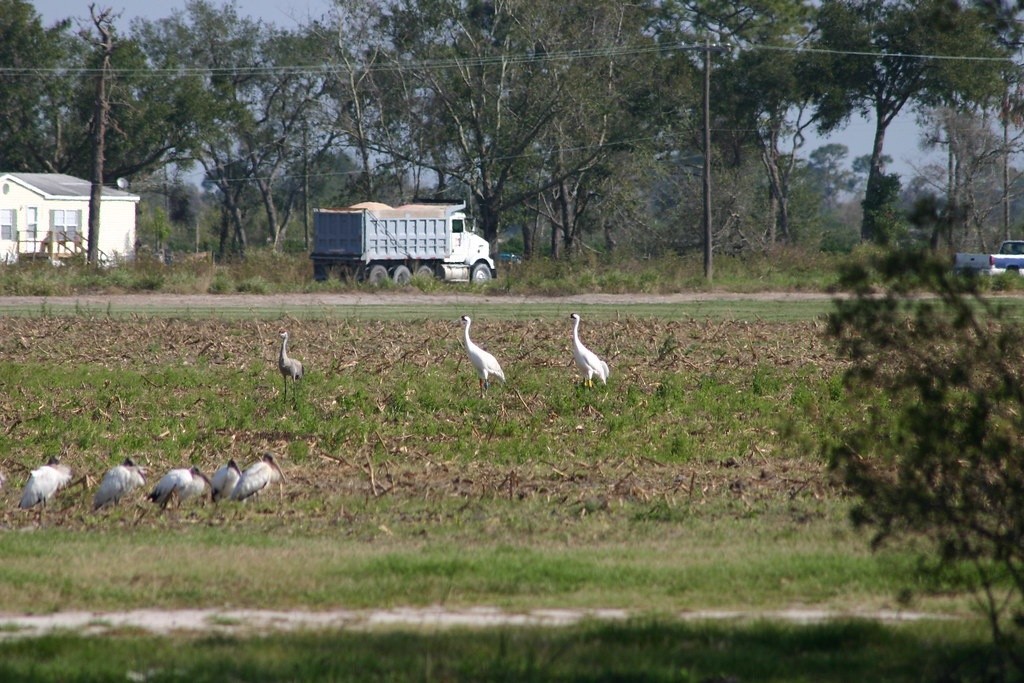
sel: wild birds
[90,452,288,517]
[570,313,610,391]
[452,315,506,398]
[17,460,72,523]
[278,328,304,403]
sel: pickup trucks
[951,238,1024,292]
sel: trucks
[308,196,498,293]
